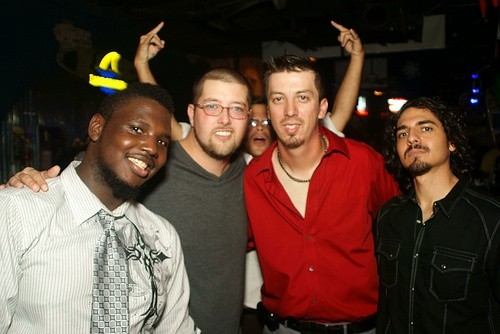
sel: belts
[277,317,375,334]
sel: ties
[90,209,129,334]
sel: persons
[372,98,500,334]
[243,54,402,334]
[0,80,201,334]
[4,66,256,334]
[133,20,365,334]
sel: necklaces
[277,132,327,183]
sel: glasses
[248,118,270,127]
[195,102,252,120]
